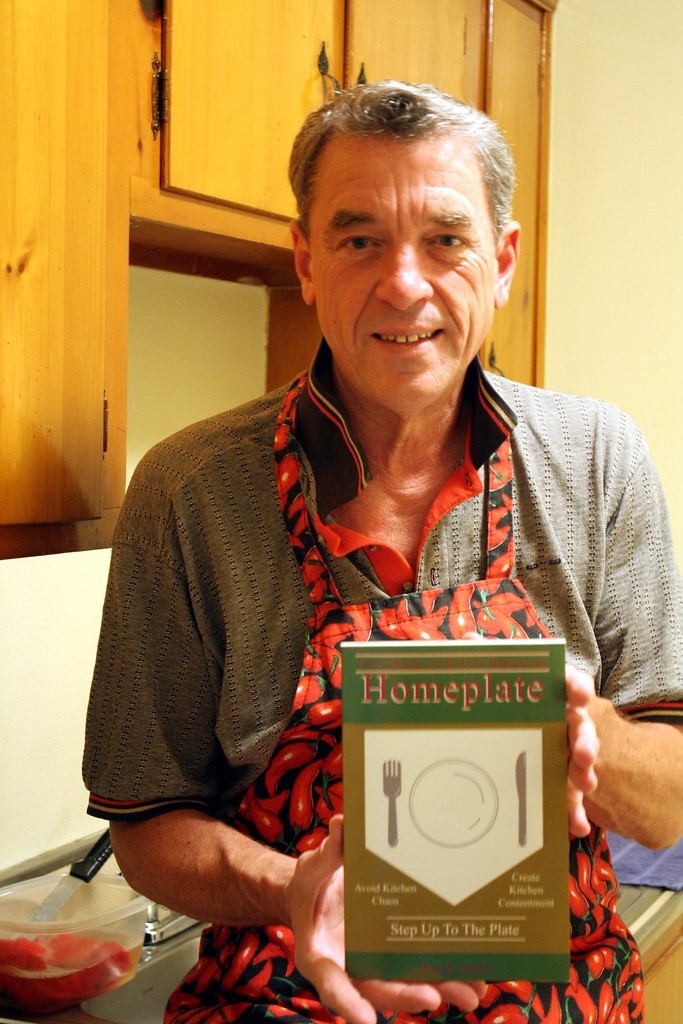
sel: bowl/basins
[0,873,152,1014]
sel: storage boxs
[0,869,154,1011]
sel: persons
[82,83,683,1024]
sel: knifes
[12,828,112,942]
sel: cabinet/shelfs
[630,910,683,1024]
[0,0,557,559]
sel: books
[340,638,571,983]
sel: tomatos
[0,932,133,1008]
